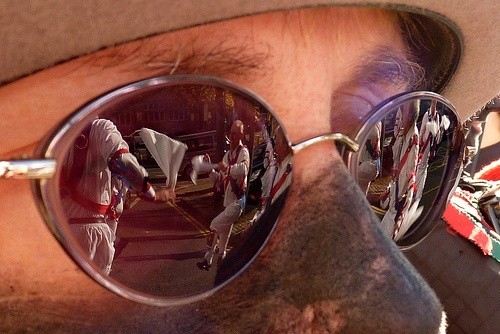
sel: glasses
[1,73,468,308]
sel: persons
[0,0,500,334]
[379,99,420,243]
[414,99,444,201]
[59,114,177,276]
[197,120,250,273]
[268,125,293,206]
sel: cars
[172,130,223,176]
[123,130,163,162]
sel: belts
[68,217,105,224]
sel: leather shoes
[197,260,211,272]
